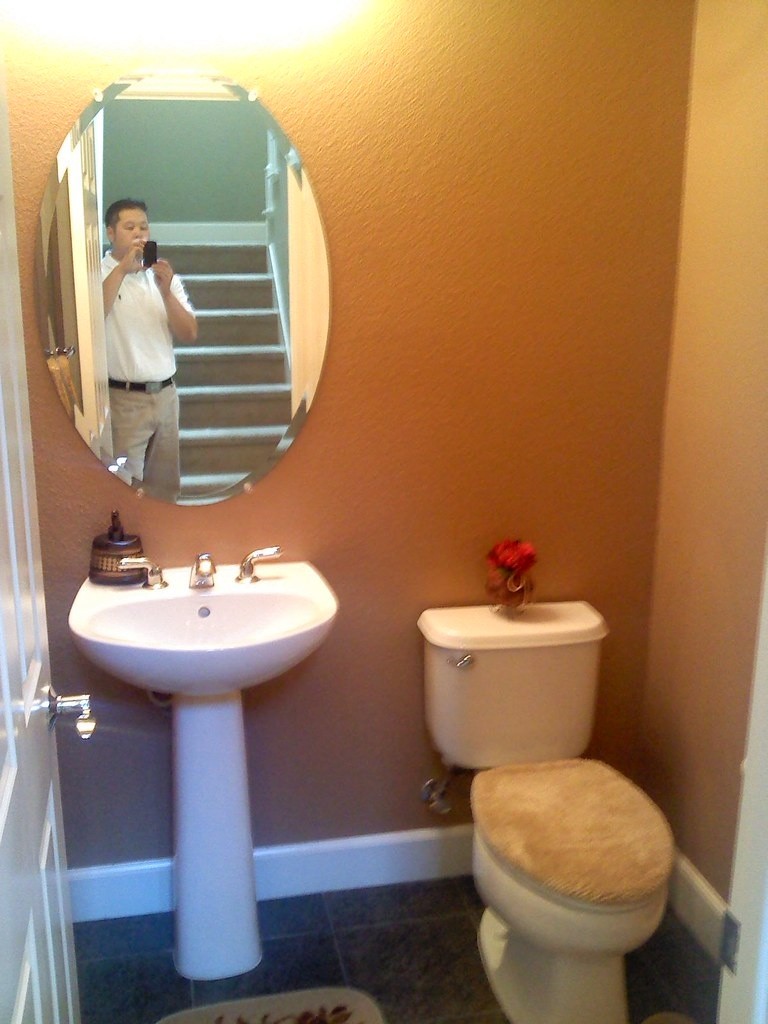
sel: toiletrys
[88,508,148,586]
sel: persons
[102,199,198,487]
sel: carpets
[153,985,387,1023]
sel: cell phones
[143,241,158,268]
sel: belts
[108,378,171,393]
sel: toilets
[414,598,678,1023]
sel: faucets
[189,552,217,589]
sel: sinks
[67,560,339,698]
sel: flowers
[487,540,535,606]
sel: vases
[485,576,535,620]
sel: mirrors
[33,72,335,506]
